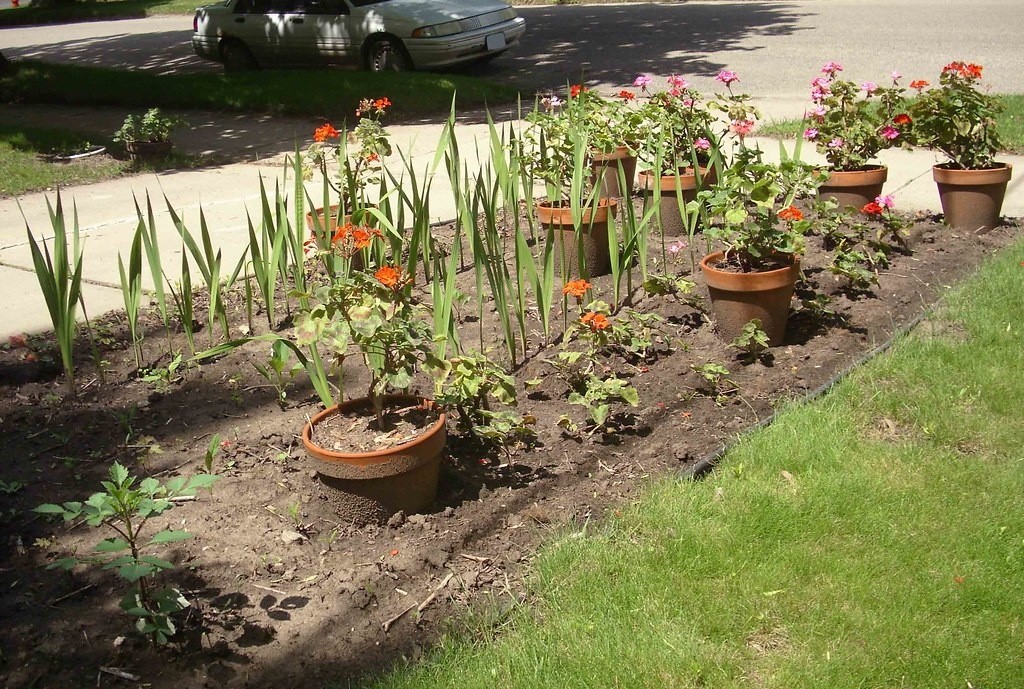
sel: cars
[191,0,528,73]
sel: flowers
[894,60,1008,167]
[801,61,903,167]
[502,66,755,196]
[304,223,450,424]
[306,97,392,199]
[685,165,813,265]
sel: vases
[306,203,378,272]
[302,395,448,514]
[932,161,1013,235]
[636,166,711,237]
[536,200,621,278]
[700,249,800,352]
[690,159,724,191]
[812,165,889,216]
[581,142,639,196]
